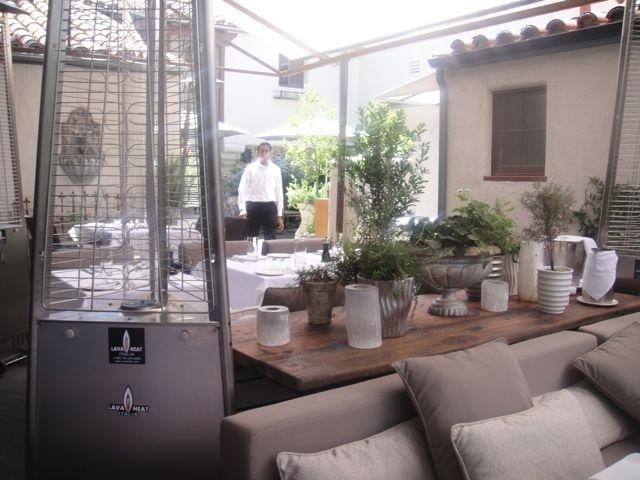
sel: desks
[69,217,202,265]
[218,293,639,391]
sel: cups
[100,246,113,273]
[246,237,258,257]
[293,237,308,267]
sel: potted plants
[286,174,317,238]
[402,187,520,316]
[536,177,575,315]
[300,266,340,326]
[160,153,184,225]
[349,158,429,338]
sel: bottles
[321,241,330,263]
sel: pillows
[448,388,607,480]
[275,415,435,479]
[391,337,534,480]
[572,321,639,426]
[525,375,635,451]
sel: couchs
[218,309,640,480]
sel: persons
[237,142,286,240]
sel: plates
[236,254,267,262]
[268,253,290,258]
[257,268,283,277]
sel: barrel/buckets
[543,239,589,287]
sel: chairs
[179,238,347,307]
[197,214,250,241]
